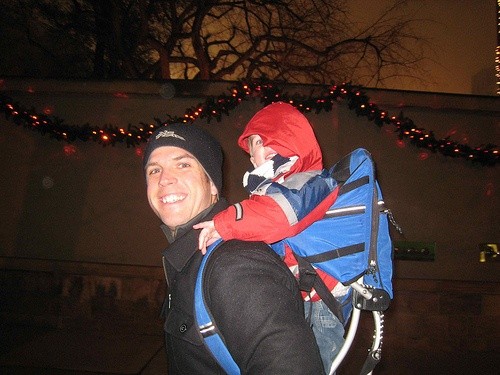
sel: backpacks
[286,148,394,312]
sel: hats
[143,123,224,200]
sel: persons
[142,122,326,375]
[192,101,353,375]
